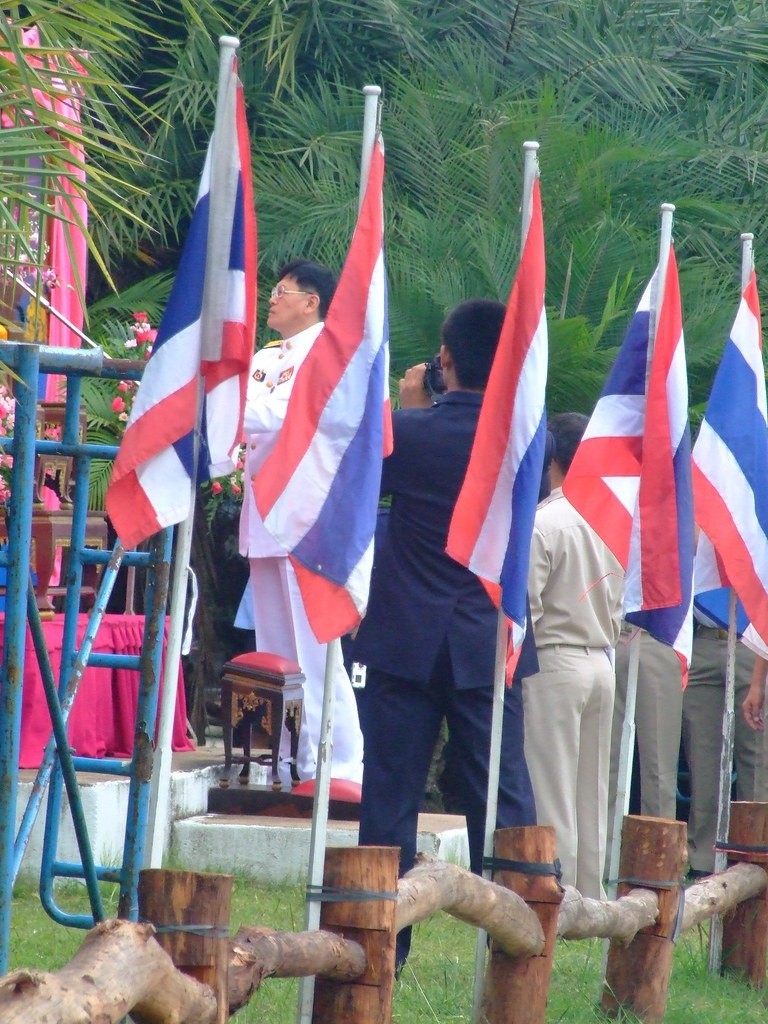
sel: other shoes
[686,866,711,880]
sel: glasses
[271,286,313,299]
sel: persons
[354,301,540,977]
[236,261,365,788]
[613,620,768,877]
[521,412,626,901]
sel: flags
[106,59,257,550]
[251,134,394,643]
[693,271,768,656]
[563,245,693,690]
[444,175,549,688]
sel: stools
[220,652,307,792]
[33,401,88,511]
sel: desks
[0,612,198,771]
[0,511,108,622]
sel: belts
[697,626,728,641]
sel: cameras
[423,354,446,395]
